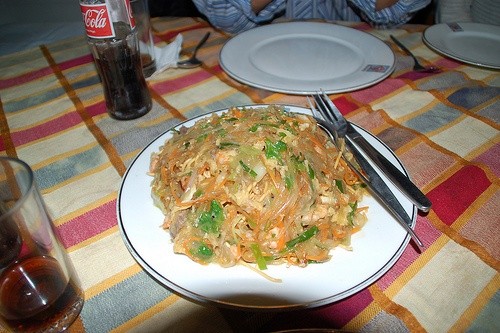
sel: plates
[218,21,397,97]
[423,21,500,70]
[116,102,418,313]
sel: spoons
[176,31,210,69]
[312,116,425,248]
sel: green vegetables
[171,111,367,270]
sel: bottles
[80,0,153,120]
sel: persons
[192,0,431,36]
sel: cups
[128,0,157,79]
[0,155,84,333]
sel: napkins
[138,33,183,81]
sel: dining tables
[0,15,500,333]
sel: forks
[389,34,441,73]
[306,87,432,213]
